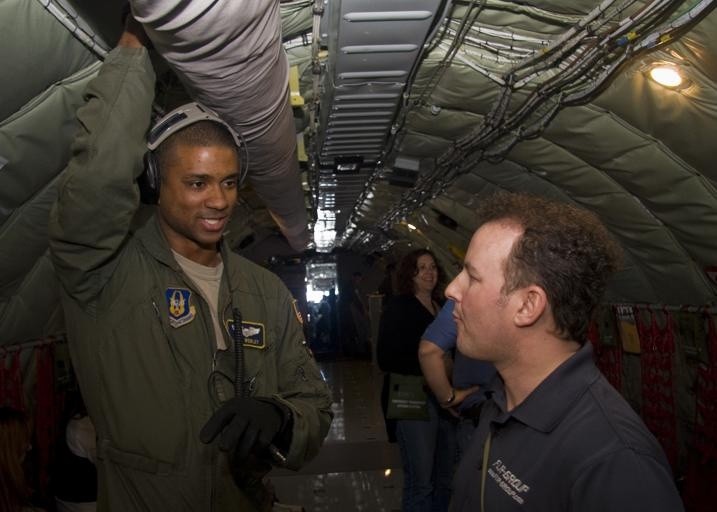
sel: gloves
[198,395,281,471]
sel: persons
[52,384,99,511]
[340,270,364,356]
[417,296,500,455]
[445,186,683,509]
[374,248,461,511]
[304,280,337,340]
[45,14,334,510]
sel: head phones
[138,101,250,206]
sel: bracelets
[439,388,456,405]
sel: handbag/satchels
[385,371,432,421]
